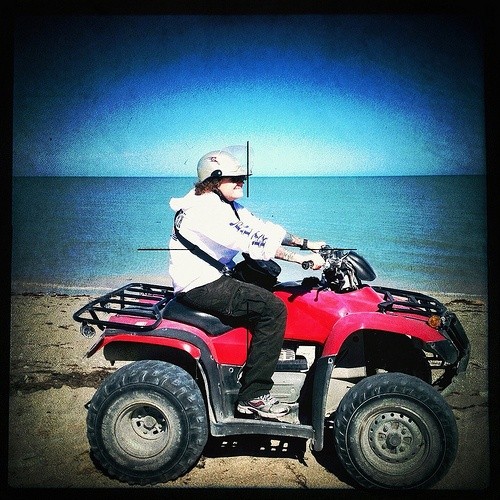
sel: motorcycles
[73,242,472,491]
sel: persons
[168,150,328,419]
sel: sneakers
[237,393,291,419]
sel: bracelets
[302,237,308,249]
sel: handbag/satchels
[174,201,282,289]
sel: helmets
[197,150,252,184]
